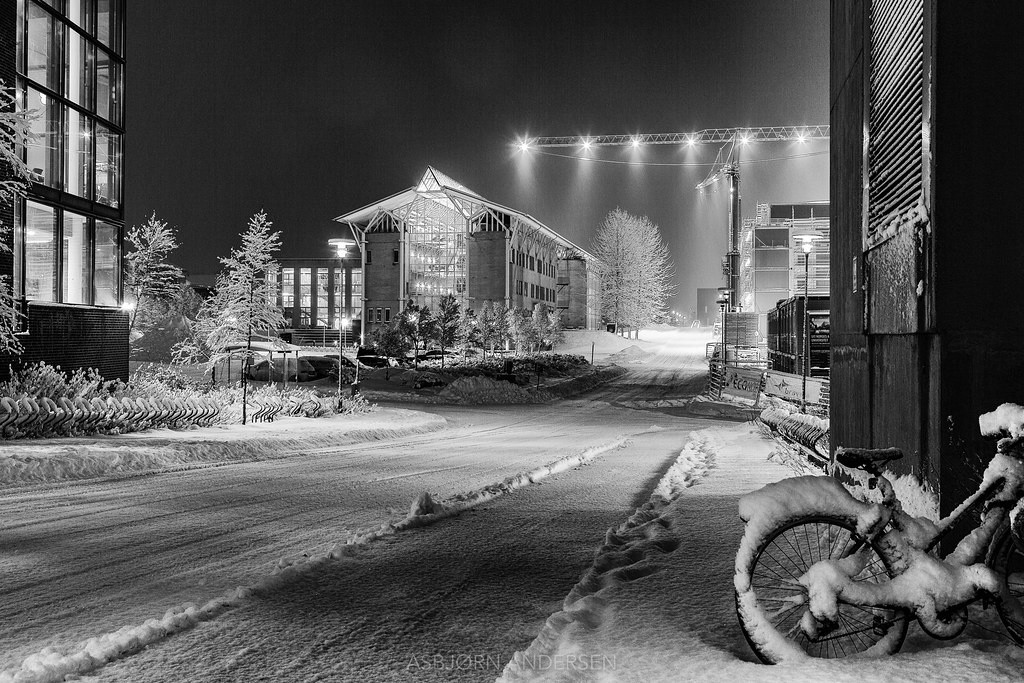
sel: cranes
[515,123,834,313]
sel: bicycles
[733,411,1023,666]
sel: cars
[246,357,318,382]
[299,348,463,380]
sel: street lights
[328,238,357,414]
[792,229,832,412]
